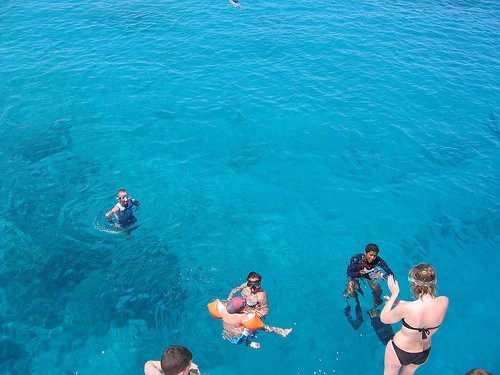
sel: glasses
[119,195,131,202]
[247,280,261,289]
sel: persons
[465,368,493,375]
[106,189,140,234]
[233,0,241,7]
[227,271,269,318]
[380,263,448,375]
[217,297,293,349]
[346,243,395,306]
[144,345,200,375]
